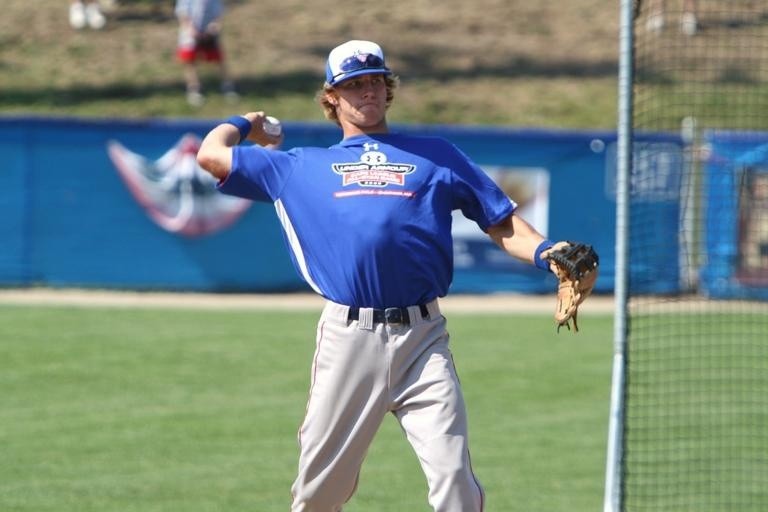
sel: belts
[349,305,428,327]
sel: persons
[196,40,612,510]
[69,1,106,33]
[174,0,243,107]
[647,1,702,39]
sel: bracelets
[221,114,251,142]
[534,239,557,272]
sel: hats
[324,41,392,88]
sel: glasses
[335,53,386,73]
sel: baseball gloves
[540,241,599,325]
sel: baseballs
[263,117,282,136]
